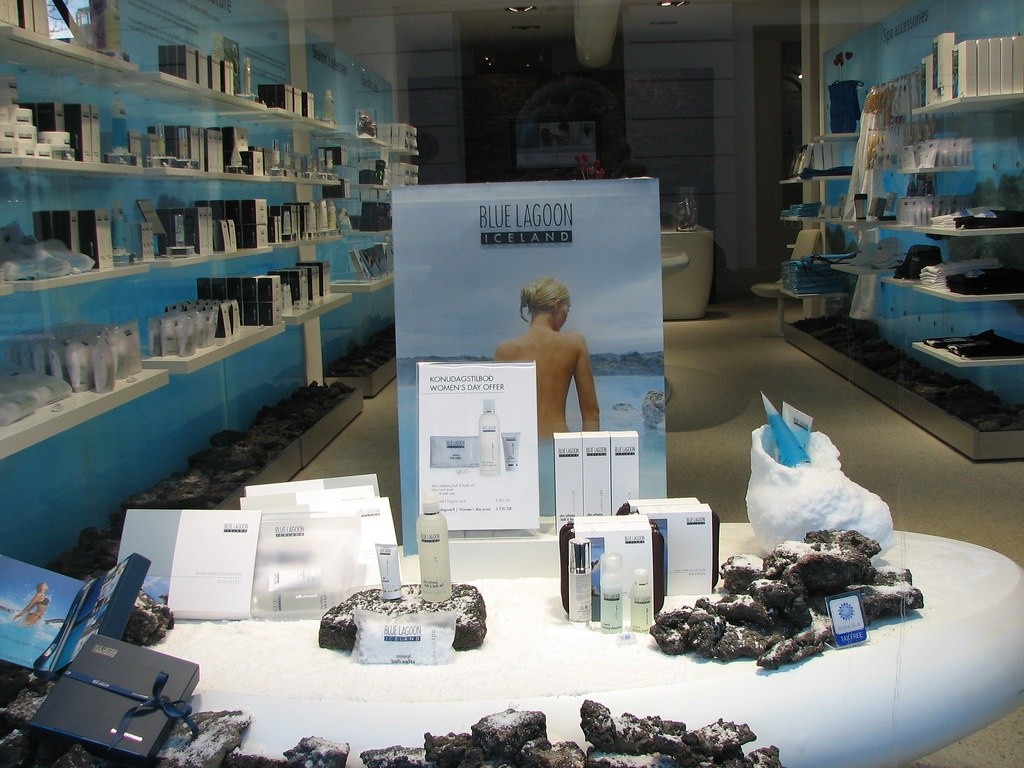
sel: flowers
[575,153,606,180]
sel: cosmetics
[780,31,1024,227]
[761,390,814,468]
[428,398,520,476]
[374,543,404,601]
[1,0,419,428]
[0,474,398,768]
[416,496,452,602]
[552,434,721,634]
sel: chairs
[753,228,821,336]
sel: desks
[140,522,1024,768]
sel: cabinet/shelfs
[776,37,1024,462]
[0,0,422,696]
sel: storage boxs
[242,275,266,302]
[243,301,258,326]
[227,276,255,301]
[212,275,239,299]
[920,32,1024,106]
[239,151,264,176]
[417,359,540,530]
[552,431,714,618]
[197,276,225,299]
[225,200,241,225]
[210,200,225,221]
[27,635,202,768]
[242,225,269,247]
[258,299,282,327]
[116,472,398,626]
[241,199,268,225]
[194,200,209,208]
[258,275,281,301]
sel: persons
[493,277,601,439]
[541,122,592,147]
[12,582,50,627]
